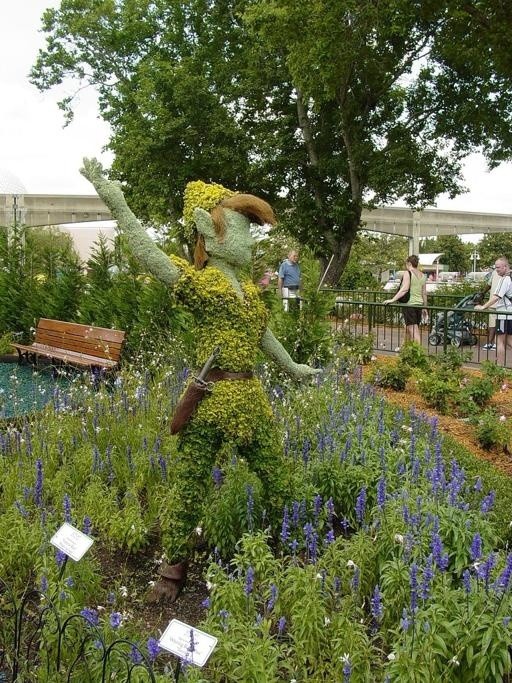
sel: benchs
[9,317,127,379]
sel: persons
[75,155,323,603]
[278,251,305,311]
[382,255,427,352]
[481,268,497,351]
[473,257,512,366]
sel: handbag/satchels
[395,269,412,304]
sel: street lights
[469,252,482,281]
[10,191,22,240]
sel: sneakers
[482,343,498,352]
[394,347,403,353]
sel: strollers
[428,291,489,348]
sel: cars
[381,270,464,291]
